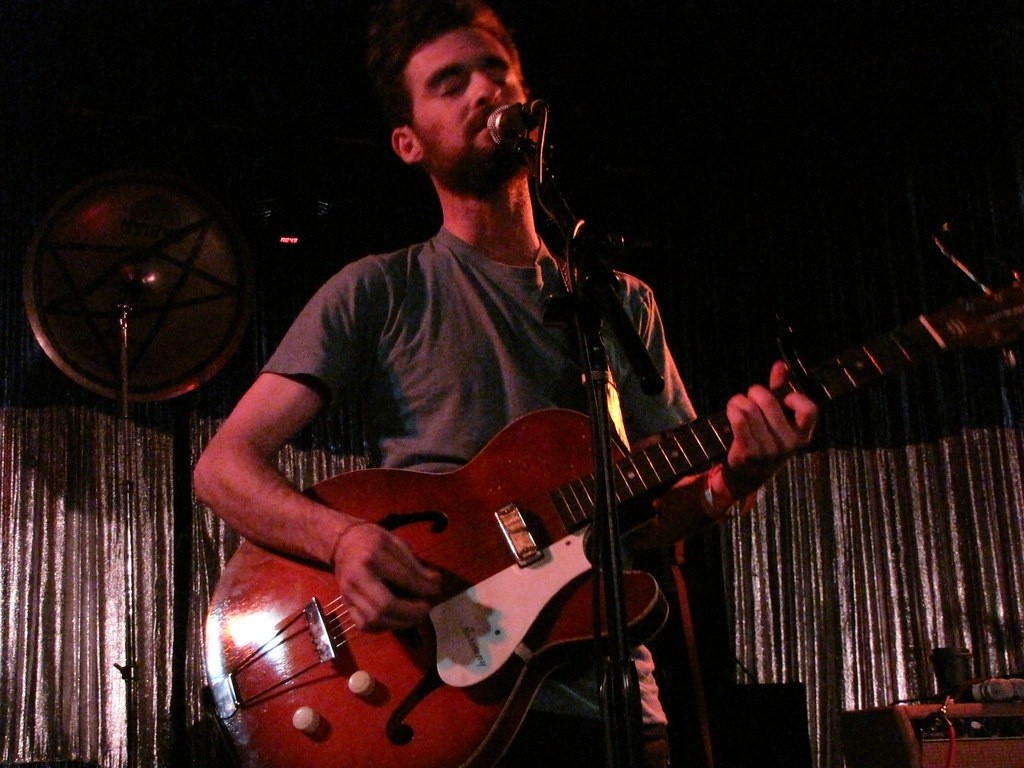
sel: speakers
[837,700,1024,768]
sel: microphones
[488,98,550,146]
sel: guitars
[196,246,1022,768]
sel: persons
[192,0,821,766]
